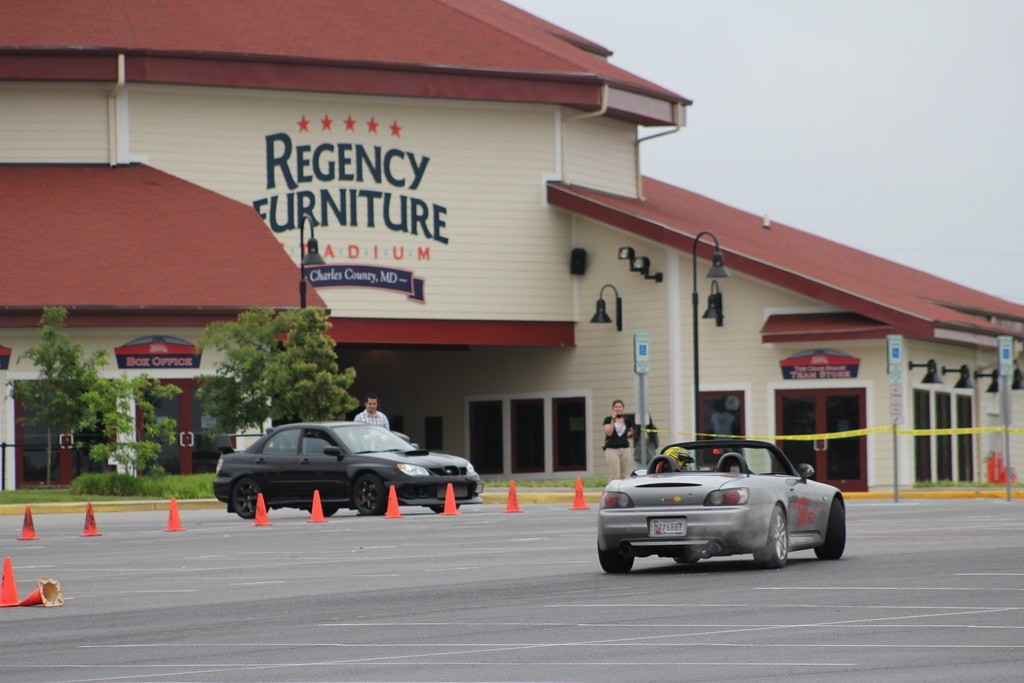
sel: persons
[353,393,389,431]
[602,400,659,484]
[663,447,692,474]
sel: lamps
[909,359,942,384]
[702,280,724,328]
[630,257,662,282]
[619,247,634,272]
[942,365,975,388]
[590,285,624,332]
[973,369,1000,393]
[1012,368,1024,390]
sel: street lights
[691,231,732,470]
[299,212,325,310]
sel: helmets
[663,446,691,469]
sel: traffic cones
[502,480,525,513]
[303,489,330,523]
[18,577,63,607]
[382,485,405,518]
[78,502,103,536]
[438,483,462,515]
[250,493,273,526]
[0,556,22,608]
[15,505,41,540]
[568,478,592,510]
[162,497,187,530]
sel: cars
[596,440,847,574]
[211,422,486,520]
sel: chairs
[713,452,754,474]
[647,454,683,473]
